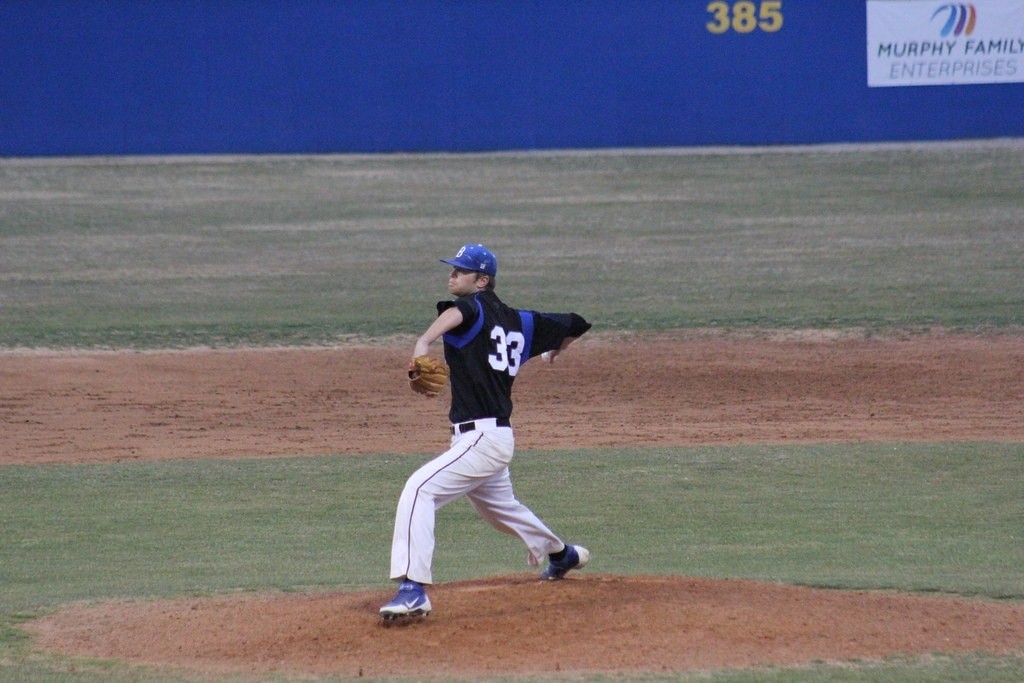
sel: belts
[448,418,512,435]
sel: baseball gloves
[407,354,449,398]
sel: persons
[377,244,592,622]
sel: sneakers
[378,581,433,620]
[540,543,590,580]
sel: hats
[440,243,497,276]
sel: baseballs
[541,351,557,363]
